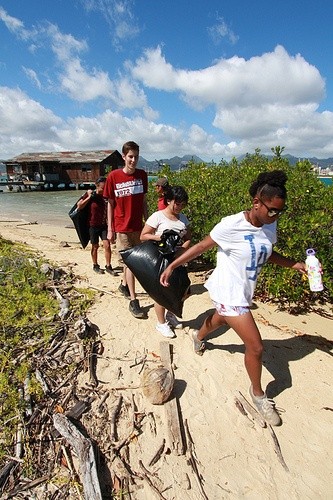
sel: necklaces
[246,211,253,225]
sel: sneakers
[188,328,205,357]
[247,385,281,426]
[118,280,131,300]
[155,310,183,338]
[104,265,118,277]
[128,299,144,319]
[92,264,105,274]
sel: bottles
[305,248,324,291]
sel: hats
[151,178,170,187]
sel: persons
[140,186,192,338]
[77,177,118,276]
[159,170,324,427]
[103,141,148,318]
[156,178,191,294]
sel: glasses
[256,195,288,218]
